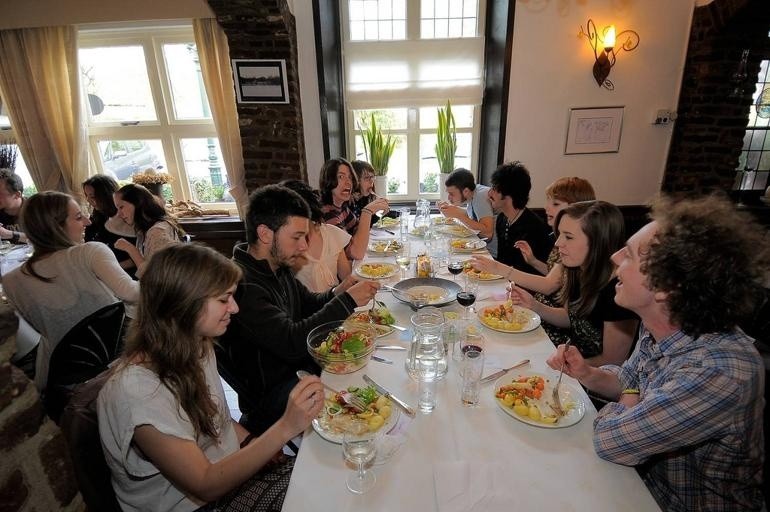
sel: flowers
[133,173,174,186]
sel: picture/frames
[564,105,625,155]
[231,58,290,105]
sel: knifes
[480,358,530,383]
[376,344,405,351]
[384,229,395,235]
[384,240,392,252]
[362,375,414,415]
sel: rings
[311,396,315,404]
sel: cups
[23,244,33,261]
[416,225,450,279]
[392,207,412,282]
[473,242,487,259]
[418,353,437,412]
[465,281,478,311]
[462,350,483,407]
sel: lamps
[578,16,640,92]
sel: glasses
[363,176,376,180]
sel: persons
[103,183,183,281]
[507,199,643,367]
[81,173,136,277]
[487,160,550,295]
[216,184,381,458]
[469,177,596,306]
[0,171,29,245]
[96,242,325,511]
[349,161,382,228]
[276,179,351,294]
[319,157,391,262]
[1,191,142,390]
[546,192,769,510]
[434,168,498,260]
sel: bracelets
[361,208,373,215]
[621,388,640,394]
[12,231,20,243]
[331,287,337,296]
[507,266,513,278]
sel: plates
[478,305,542,334]
[461,270,504,281]
[495,370,585,426]
[309,396,399,443]
[351,311,397,338]
[368,240,405,253]
[357,260,400,278]
[453,239,487,251]
[431,216,457,225]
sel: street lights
[182,37,226,197]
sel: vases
[142,183,166,197]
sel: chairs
[67,399,122,511]
[43,300,131,439]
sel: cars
[97,134,159,178]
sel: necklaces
[507,209,521,226]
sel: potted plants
[433,98,459,203]
[356,111,398,200]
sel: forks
[370,188,382,199]
[297,370,369,412]
[371,295,387,308]
[553,333,571,418]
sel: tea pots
[406,306,450,381]
[413,198,432,229]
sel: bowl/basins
[392,278,463,306]
[304,320,378,376]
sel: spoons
[368,293,375,318]
[507,280,514,313]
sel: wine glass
[0,240,11,265]
[460,334,487,379]
[376,209,387,229]
[457,286,476,323]
[447,258,464,281]
[341,423,377,494]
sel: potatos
[488,319,522,330]
[370,266,393,276]
[368,395,391,430]
[504,393,541,421]
